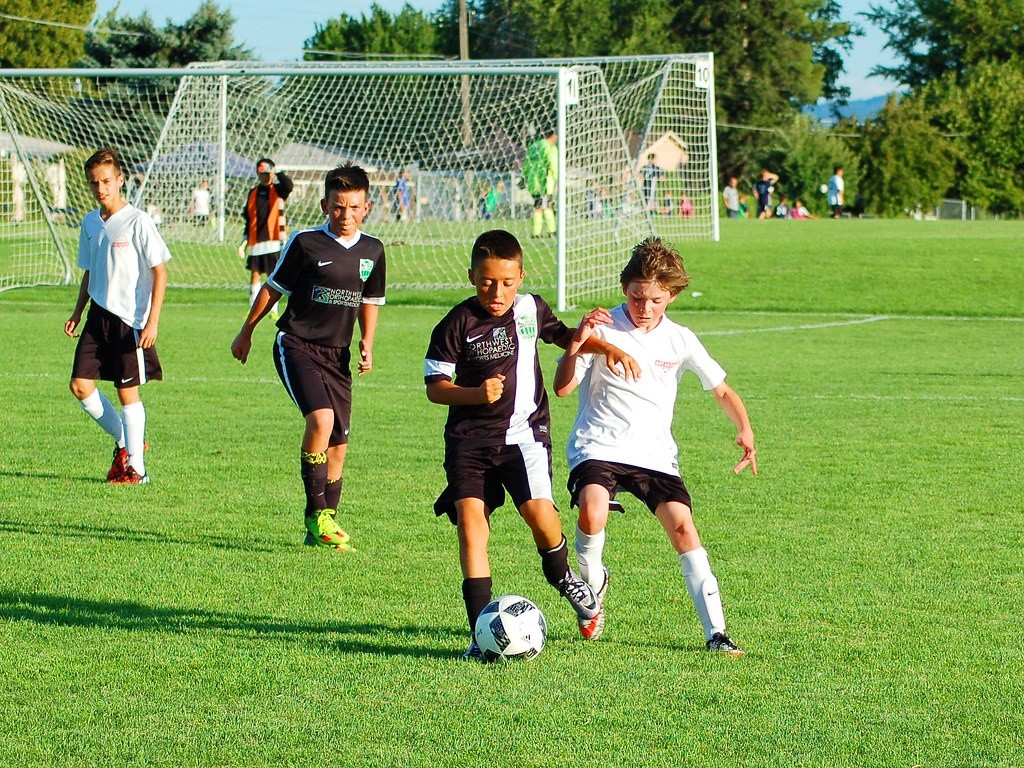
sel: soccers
[475,595,547,663]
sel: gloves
[259,162,281,173]
[238,239,249,260]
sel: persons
[553,237,757,656]
[238,159,293,319]
[424,229,641,658]
[829,167,844,218]
[231,161,386,552]
[479,184,499,220]
[129,171,161,227]
[189,181,210,228]
[585,153,692,219]
[722,171,818,219]
[64,150,171,484]
[394,169,412,224]
[523,131,558,238]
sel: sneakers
[706,632,745,657]
[303,533,355,552]
[107,439,148,481]
[553,566,601,619]
[305,508,349,544]
[577,566,609,640]
[109,465,150,485]
[462,641,483,660]
